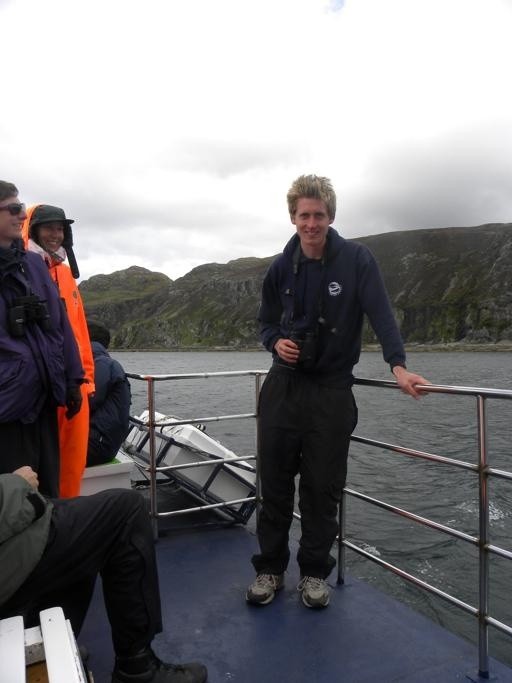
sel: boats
[0,366,511,682]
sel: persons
[0,179,86,501]
[18,204,97,497]
[1,461,208,683]
[85,318,132,467]
[242,174,432,610]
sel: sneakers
[245,573,284,604]
[112,660,208,683]
[296,576,331,609]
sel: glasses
[0,202,25,215]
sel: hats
[29,205,74,226]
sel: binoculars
[8,295,52,337]
[286,330,316,373]
